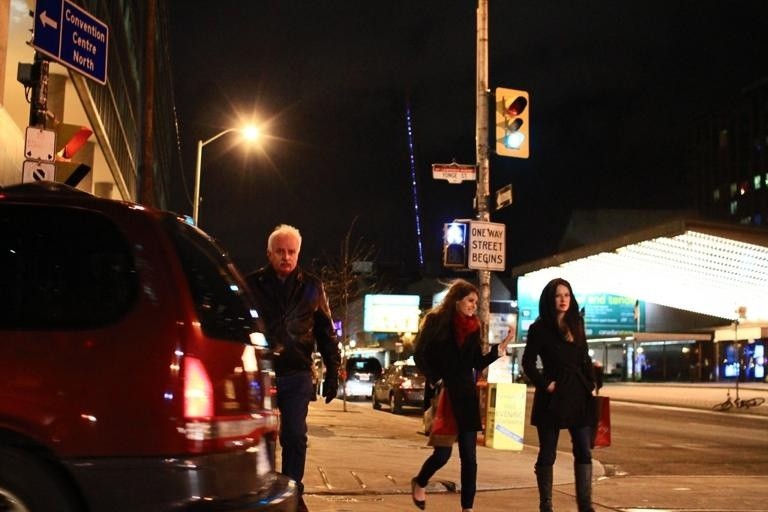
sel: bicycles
[713,393,765,414]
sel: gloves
[323,371,340,404]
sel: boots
[534,465,554,511]
[574,462,594,512]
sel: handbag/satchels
[423,386,458,448]
[591,397,610,450]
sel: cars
[319,357,382,401]
[2,181,300,512]
[371,364,425,413]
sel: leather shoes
[411,477,426,511]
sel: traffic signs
[431,163,476,184]
[30,0,110,86]
[467,220,506,272]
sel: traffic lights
[442,222,466,268]
[495,86,529,160]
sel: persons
[411,279,516,512]
[240,223,341,484]
[521,278,602,512]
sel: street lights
[193,119,263,228]
